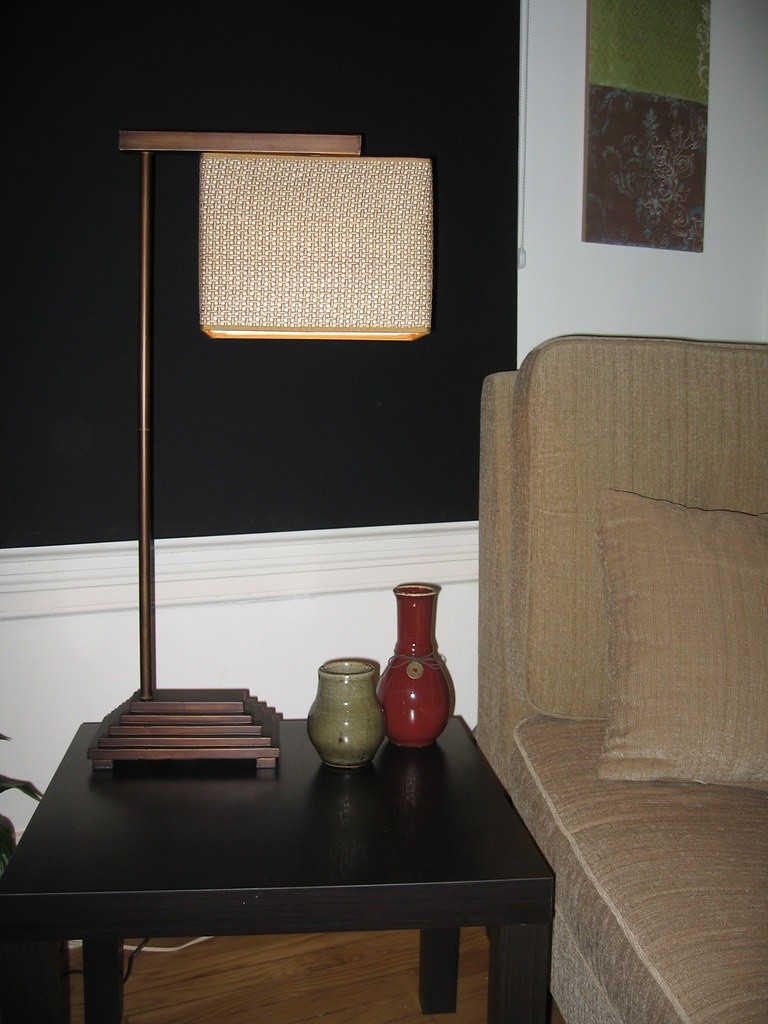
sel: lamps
[87,132,431,777]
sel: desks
[0,723,557,1024]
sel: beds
[477,327,768,1024]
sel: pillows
[596,487,768,792]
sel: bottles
[307,660,387,768]
[376,585,450,748]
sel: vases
[306,660,385,768]
[378,582,455,750]
